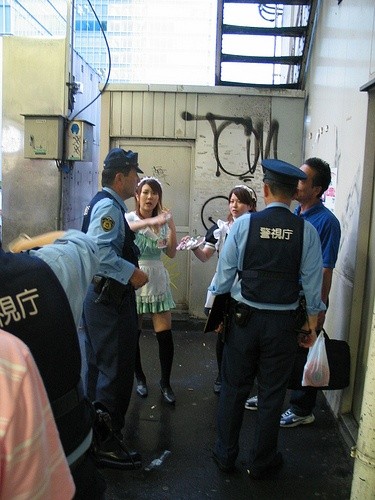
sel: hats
[104,148,143,173]
[261,159,307,186]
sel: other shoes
[213,376,222,392]
[92,444,143,465]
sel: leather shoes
[136,378,148,397]
[216,454,234,472]
[249,456,282,479]
[160,379,176,404]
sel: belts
[92,275,103,284]
[50,375,85,418]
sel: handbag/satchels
[286,326,350,390]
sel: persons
[125,176,177,403]
[0,229,111,500]
[244,157,341,428]
[182,185,258,393]
[205,158,327,480]
[82,150,150,465]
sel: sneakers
[280,409,315,427]
[245,395,259,410]
